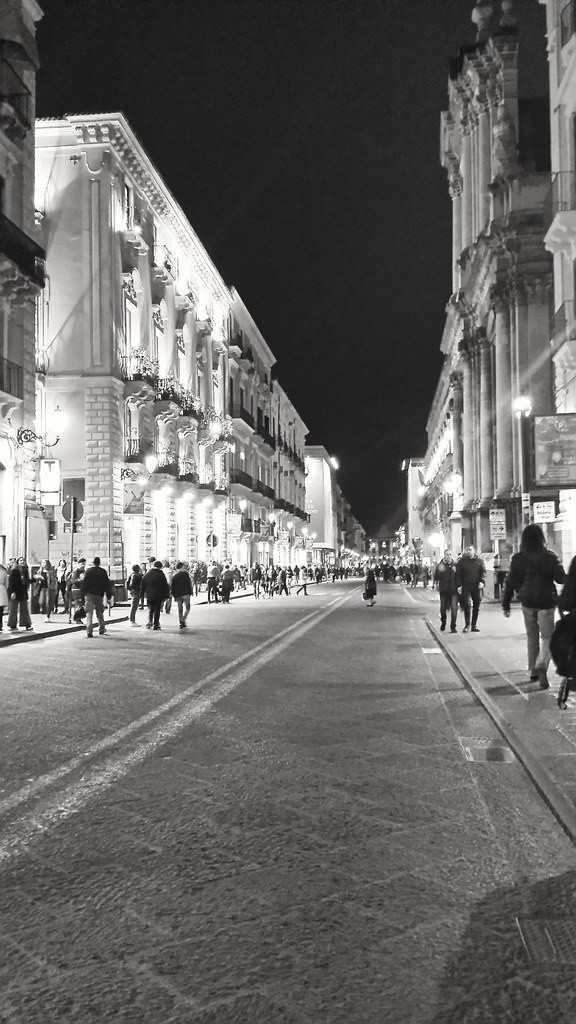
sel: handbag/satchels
[363,589,371,600]
[73,601,86,621]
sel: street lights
[239,500,317,566]
[511,396,533,527]
[340,546,360,566]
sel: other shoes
[441,622,446,631]
[180,617,187,629]
[130,622,142,627]
[153,626,162,630]
[463,625,470,632]
[146,623,153,627]
[534,667,549,689]
[44,618,52,623]
[451,627,457,633]
[471,626,480,632]
[26,625,34,631]
[11,628,23,633]
[87,631,93,637]
[77,620,83,624]
[529,671,539,682]
[99,629,107,634]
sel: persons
[0,544,487,637]
[501,523,568,689]
